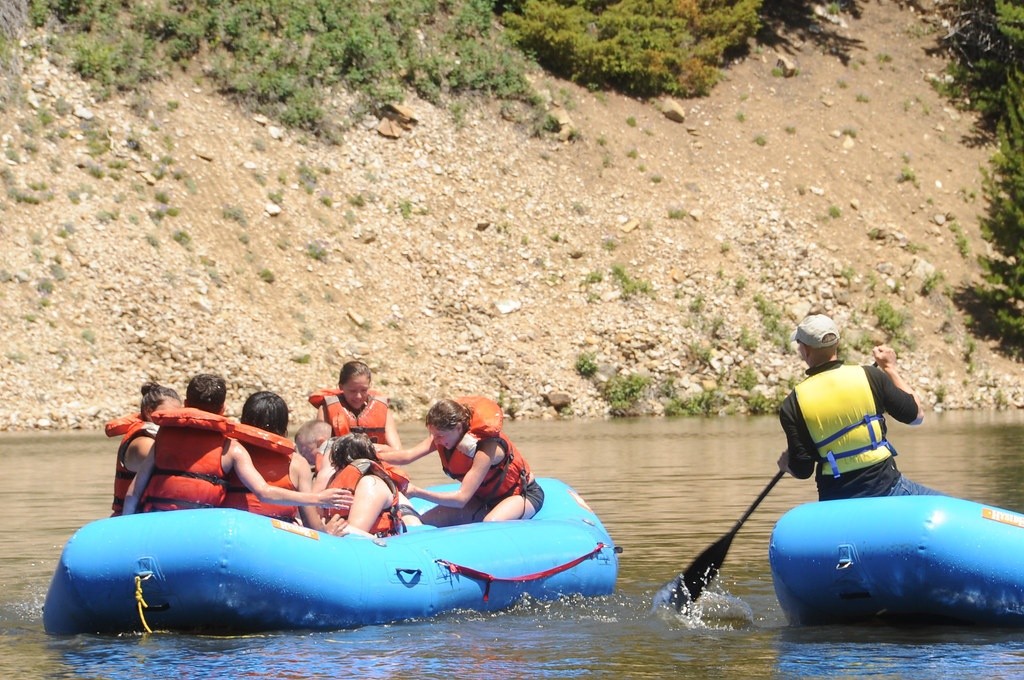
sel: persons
[224,392,350,537]
[777,314,943,502]
[309,362,402,452]
[377,398,544,527]
[295,420,410,537]
[123,374,355,515]
[105,382,182,511]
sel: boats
[769,494,1024,627]
[42,477,623,635]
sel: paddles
[664,361,879,613]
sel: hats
[789,314,840,349]
